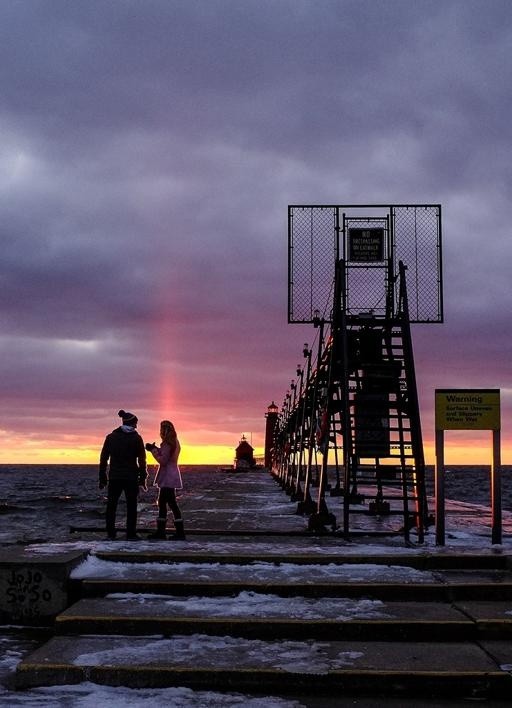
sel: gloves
[99,478,107,490]
[138,479,147,491]
[146,442,156,452]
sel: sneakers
[100,534,144,541]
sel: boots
[147,518,168,540]
[169,518,185,540]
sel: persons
[145,419,186,541]
[99,409,149,539]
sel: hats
[118,410,138,426]
